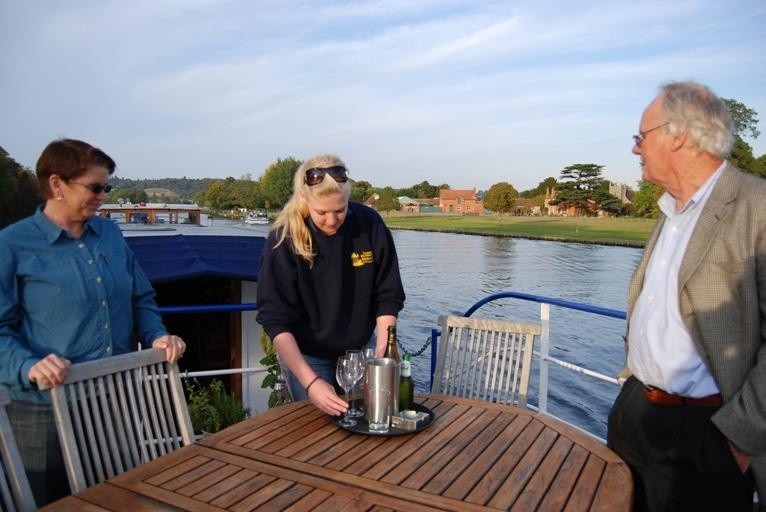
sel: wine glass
[335,349,364,428]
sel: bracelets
[305,376,321,396]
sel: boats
[244,212,273,224]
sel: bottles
[382,322,415,411]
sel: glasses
[70,181,112,194]
[304,165,347,186]
[633,123,668,147]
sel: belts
[644,388,719,407]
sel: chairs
[429,315,542,408]
[37,347,197,495]
[0,385,39,511]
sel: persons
[256,156,405,418]
[607,82,766,512]
[0,140,188,512]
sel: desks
[98,203,201,226]
[35,391,634,510]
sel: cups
[367,387,391,435]
[365,358,398,425]
[363,346,380,363]
